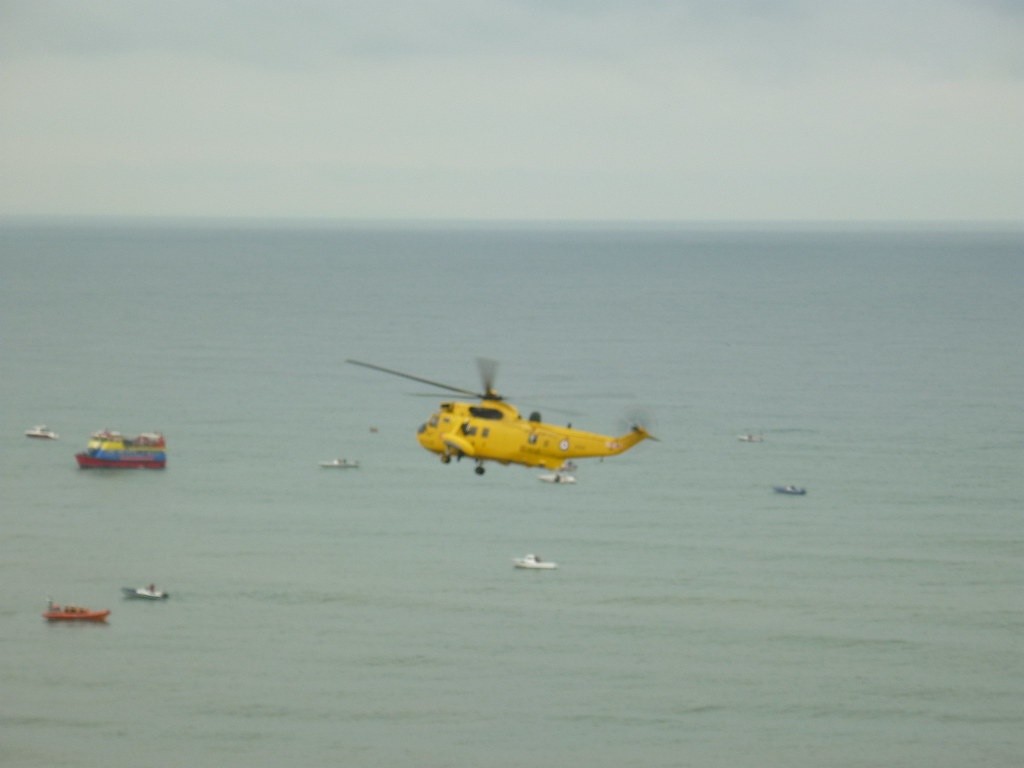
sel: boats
[539,473,576,483]
[74,429,166,468]
[122,583,169,600]
[513,554,557,569]
[322,459,359,468]
[772,484,806,496]
[42,596,111,622]
[25,424,58,439]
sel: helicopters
[345,354,661,476]
